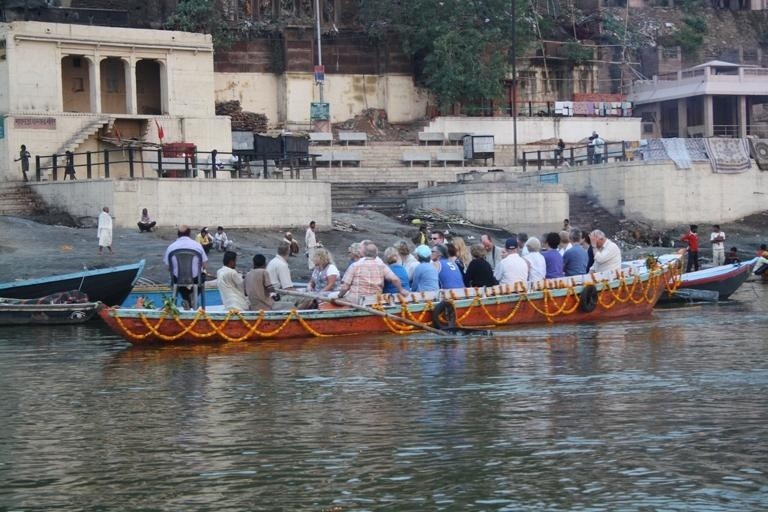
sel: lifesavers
[432,301,456,329]
[581,285,598,313]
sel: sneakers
[181,300,189,311]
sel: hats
[415,245,431,257]
[505,238,518,249]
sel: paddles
[665,289,719,302]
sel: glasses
[430,238,440,240]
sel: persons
[494,220,621,286]
[680,224,699,273]
[137,208,156,233]
[337,239,420,298]
[163,224,208,310]
[65,151,76,180]
[305,221,318,248]
[710,224,726,267]
[243,254,296,310]
[208,150,222,170]
[304,248,343,296]
[217,251,250,312]
[556,133,606,167]
[97,207,115,255]
[266,241,299,302]
[14,145,31,182]
[195,226,233,255]
[724,244,768,276]
[229,152,246,169]
[283,231,299,256]
[405,223,502,289]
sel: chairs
[169,248,205,310]
